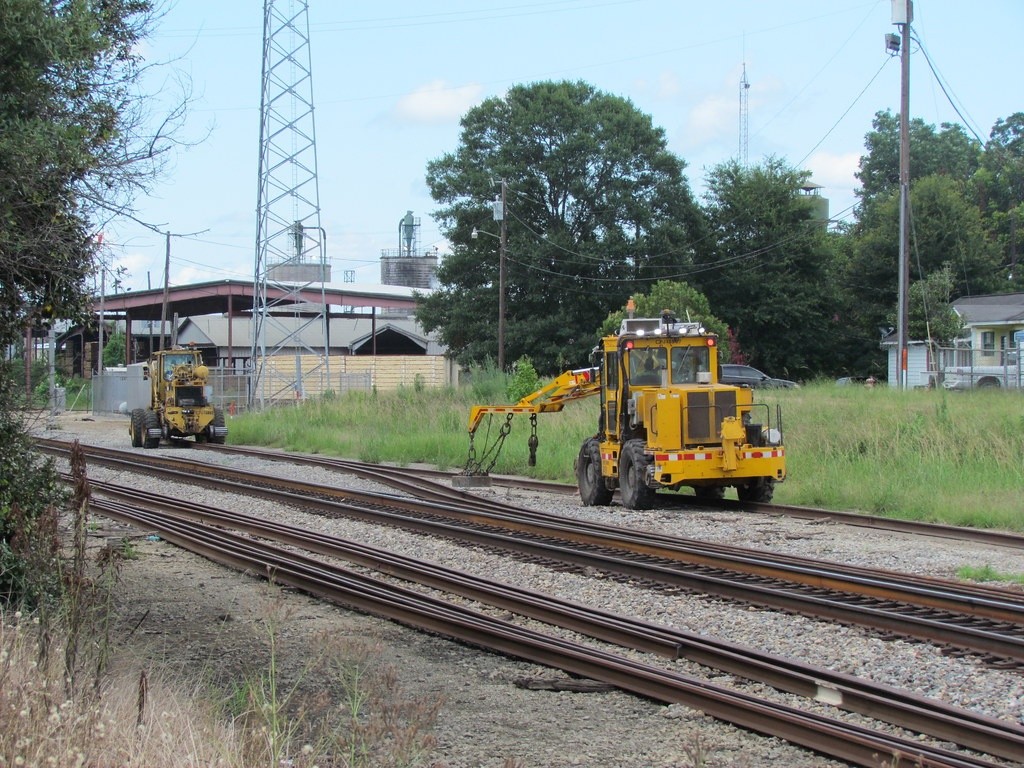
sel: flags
[83,234,105,253]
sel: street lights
[471,226,506,372]
[885,33,909,391]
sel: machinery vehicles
[452,294,786,510]
[128,342,228,449]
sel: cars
[720,364,798,389]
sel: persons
[634,357,662,386]
[166,359,178,374]
